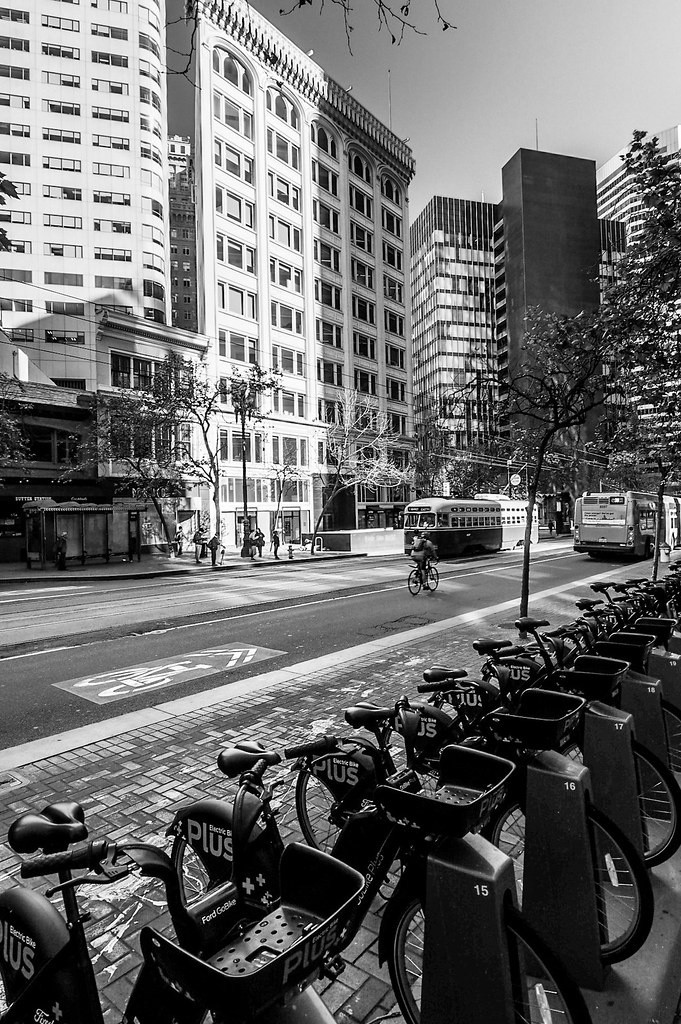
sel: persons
[255,528,265,557]
[209,533,226,566]
[176,526,187,555]
[410,532,439,590]
[58,531,69,571]
[548,520,554,534]
[272,531,281,559]
[196,527,204,563]
[249,530,260,561]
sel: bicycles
[0,757,368,1024]
[294,558,681,966]
[163,694,593,1024]
[407,558,440,595]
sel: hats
[61,531,67,536]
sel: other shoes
[212,563,218,566]
[423,585,429,589]
[196,561,202,563]
[251,558,256,560]
[275,557,280,560]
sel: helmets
[421,532,429,539]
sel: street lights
[231,379,256,557]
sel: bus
[398,463,539,558]
[572,478,681,561]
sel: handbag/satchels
[207,540,211,548]
[250,538,256,546]
[256,537,265,546]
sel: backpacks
[412,536,427,551]
[175,532,182,541]
